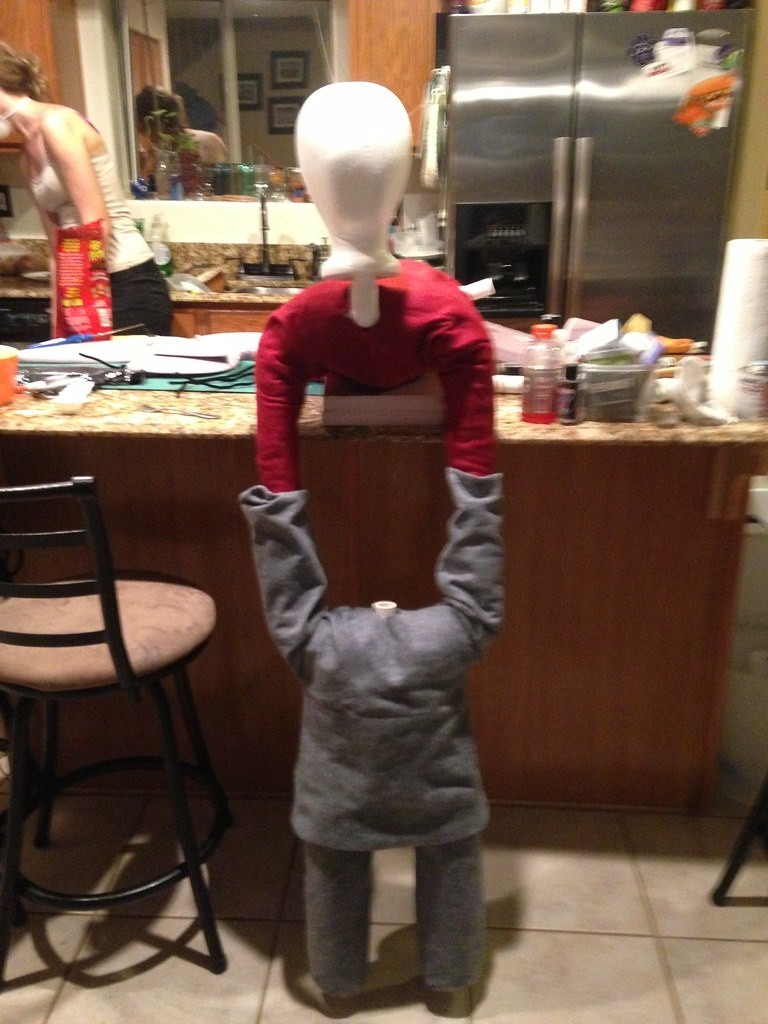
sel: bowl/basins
[52,395,86,413]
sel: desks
[0,354,766,814]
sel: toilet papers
[706,238,768,419]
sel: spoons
[141,405,219,420]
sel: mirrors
[111,1,344,205]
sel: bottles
[521,324,560,424]
[154,157,303,201]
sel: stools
[710,779,767,905]
[0,476,234,974]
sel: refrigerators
[435,7,759,358]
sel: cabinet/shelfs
[167,301,294,339]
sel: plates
[21,270,52,282]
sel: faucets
[224,192,308,282]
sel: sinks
[235,285,307,296]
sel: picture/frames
[236,74,263,110]
[267,96,304,135]
[270,49,310,90]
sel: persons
[240,78,504,996]
[135,80,229,184]
[0,39,173,337]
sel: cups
[1,346,19,406]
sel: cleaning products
[134,212,174,279]
